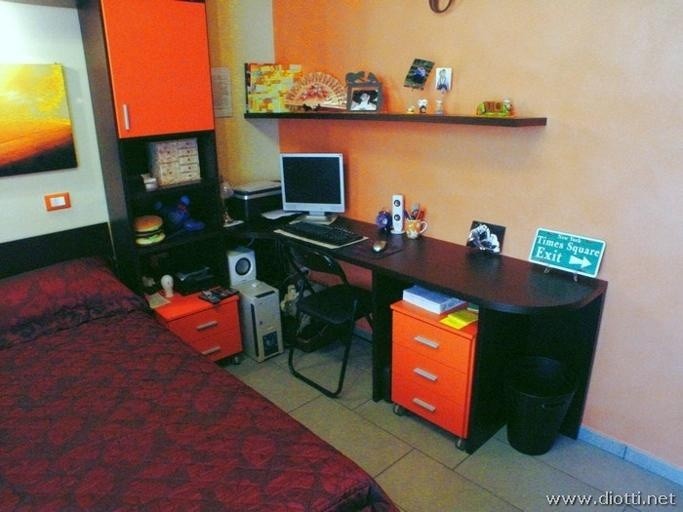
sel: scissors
[412,209,424,220]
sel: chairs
[268,225,376,402]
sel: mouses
[371,239,388,253]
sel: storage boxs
[226,175,283,224]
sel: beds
[0,220,406,511]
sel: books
[402,281,464,314]
[175,258,215,294]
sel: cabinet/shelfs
[75,0,232,297]
[386,290,484,450]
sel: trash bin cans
[503,375,583,454]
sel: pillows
[0,252,140,351]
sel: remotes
[200,289,220,305]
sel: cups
[405,218,427,240]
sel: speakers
[390,193,406,235]
[224,246,257,287]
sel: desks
[256,214,607,457]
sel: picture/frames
[344,82,384,113]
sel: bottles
[502,94,512,116]
[434,97,444,114]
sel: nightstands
[146,280,245,364]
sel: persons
[352,93,377,112]
[436,70,449,91]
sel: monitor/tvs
[279,152,346,226]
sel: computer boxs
[230,278,284,364]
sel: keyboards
[279,221,363,247]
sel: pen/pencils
[403,207,424,221]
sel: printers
[226,180,302,222]
